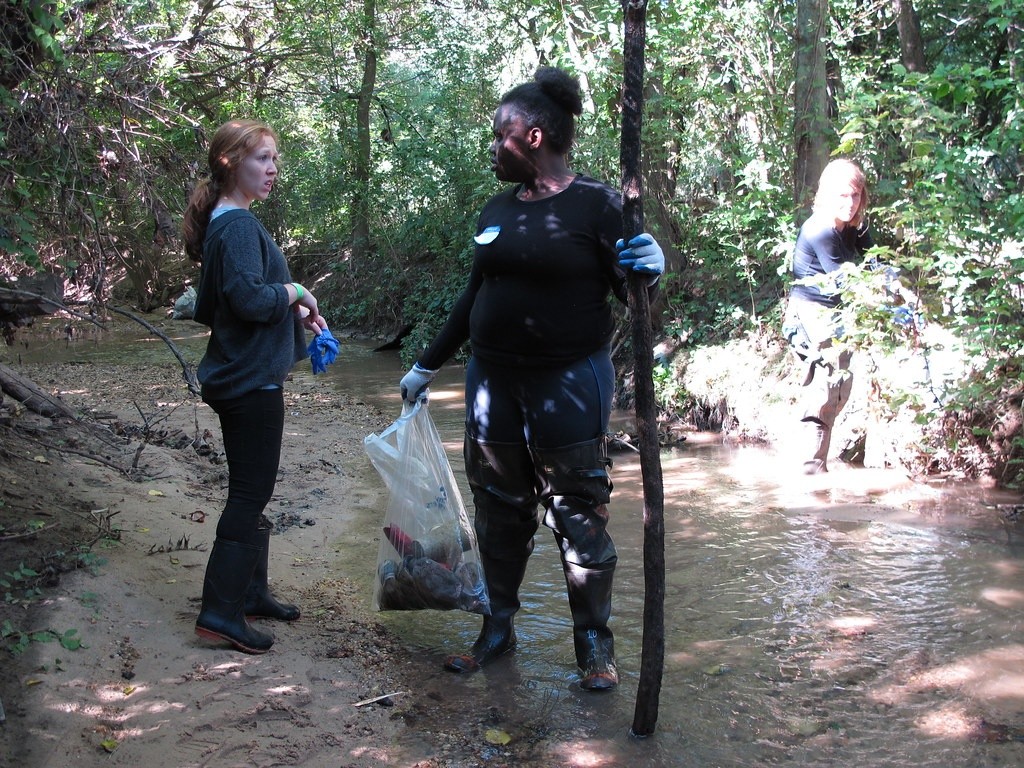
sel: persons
[400,64,666,692]
[782,158,930,475]
[183,120,340,656]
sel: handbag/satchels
[363,387,492,615]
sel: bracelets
[291,282,304,301]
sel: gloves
[615,233,666,276]
[399,361,439,404]
[305,328,340,375]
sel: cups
[410,520,477,559]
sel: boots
[562,559,620,692]
[443,578,521,673]
[240,526,301,621]
[194,537,275,654]
